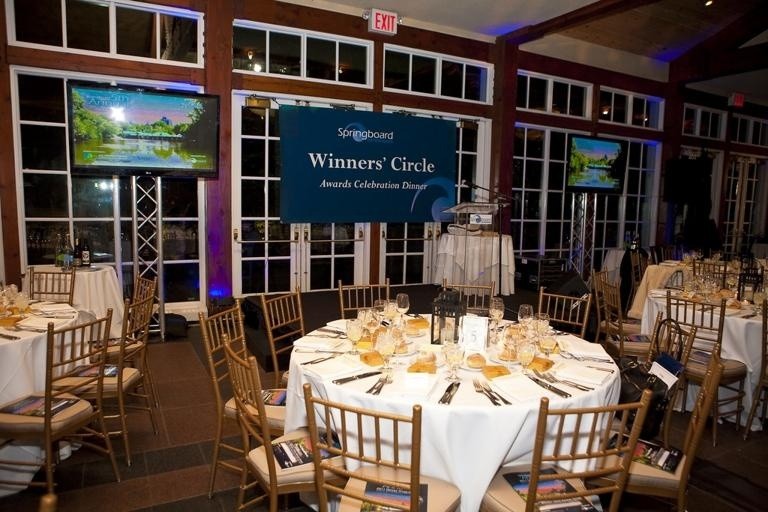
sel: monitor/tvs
[662,156,712,207]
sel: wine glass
[753,293,764,318]
[540,335,557,364]
[518,345,534,375]
[489,297,504,334]
[518,304,533,334]
[445,347,465,379]
[396,294,409,325]
[374,299,388,330]
[535,313,550,338]
[346,321,363,354]
[548,362,549,364]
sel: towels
[294,336,350,353]
[568,343,610,360]
[19,316,67,331]
[710,306,739,315]
[328,319,356,331]
[392,370,434,398]
[556,363,611,385]
[41,303,76,314]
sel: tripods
[473,187,518,315]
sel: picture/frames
[277,106,458,222]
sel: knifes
[528,376,571,399]
[296,356,341,365]
[332,366,383,385]
[527,373,580,399]
[438,381,461,403]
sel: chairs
[742,299,768,441]
[40,493,60,512]
[740,263,764,292]
[52,287,160,468]
[610,311,697,446]
[29,266,76,305]
[626,250,641,312]
[585,344,724,512]
[600,274,651,362]
[338,278,389,318]
[593,267,626,343]
[693,259,727,288]
[709,248,723,259]
[303,384,460,512]
[0,308,120,494]
[443,278,495,316]
[662,245,676,261]
[199,299,287,500]
[537,287,593,338]
[88,271,157,415]
[478,389,653,512]
[666,289,748,448]
[261,286,305,388]
[741,256,759,271]
[221,333,348,512]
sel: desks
[434,231,515,297]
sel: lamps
[456,118,479,128]
[245,94,271,109]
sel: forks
[559,350,615,374]
[317,323,351,340]
[533,368,594,391]
[366,371,390,396]
[473,381,511,406]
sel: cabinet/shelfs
[538,258,567,291]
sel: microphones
[461,179,477,189]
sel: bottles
[73,239,82,267]
[81,240,91,267]
[63,234,73,270]
[55,234,64,267]
